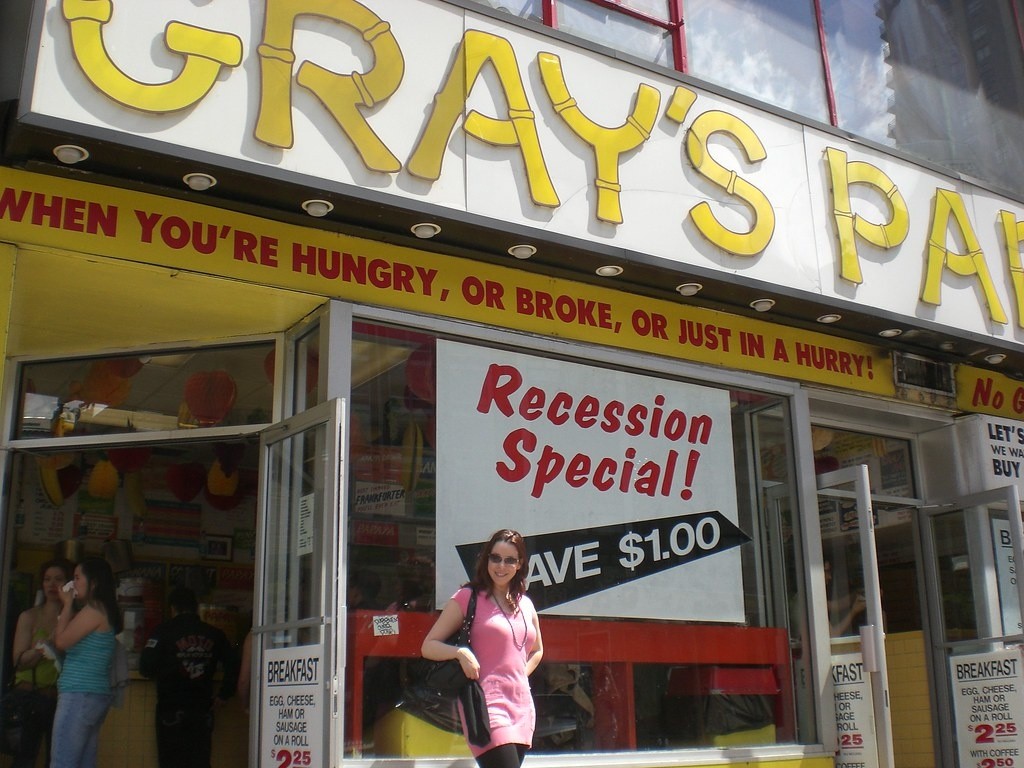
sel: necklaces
[492,592,528,647]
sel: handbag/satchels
[0,651,45,757]
[426,589,478,699]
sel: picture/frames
[204,533,234,562]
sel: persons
[344,565,387,727]
[238,630,311,699]
[140,588,241,768]
[12,561,77,768]
[208,571,214,588]
[365,556,456,757]
[49,557,124,768]
[209,542,225,555]
[546,665,619,753]
[788,557,866,639]
[420,528,543,768]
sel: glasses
[487,553,522,564]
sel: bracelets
[57,616,68,622]
